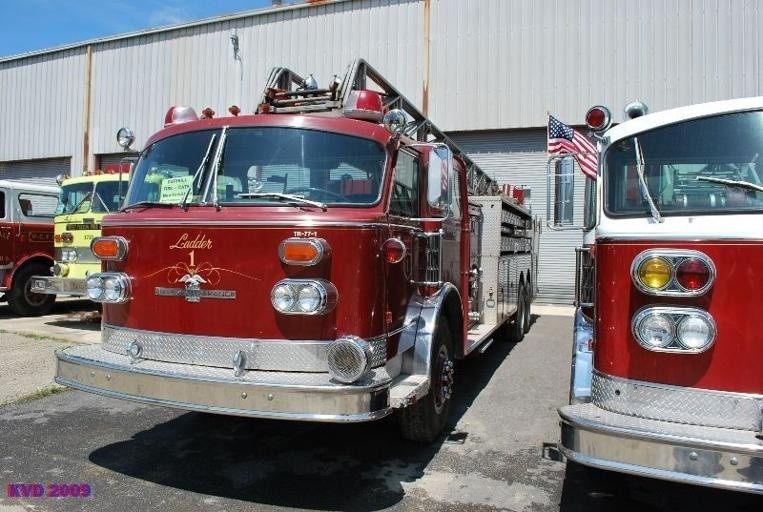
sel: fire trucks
[554,95,762,496]
[52,54,541,445]
[30,162,132,302]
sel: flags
[547,113,600,182]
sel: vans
[0,178,62,317]
